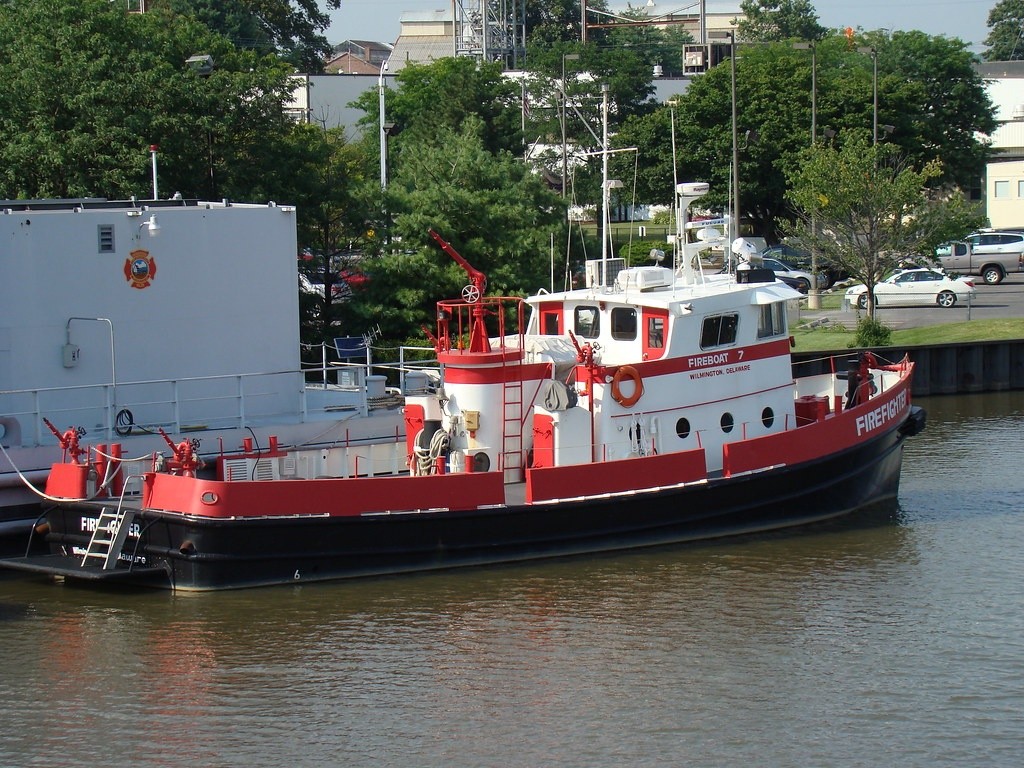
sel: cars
[299,246,370,305]
[762,256,829,294]
[844,267,976,310]
[760,245,852,290]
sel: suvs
[927,231,1024,256]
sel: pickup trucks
[896,241,1024,285]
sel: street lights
[792,40,824,311]
[707,30,741,240]
[377,60,397,254]
[601,178,624,292]
[185,53,219,199]
[856,44,896,282]
[562,52,580,226]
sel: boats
[1,228,929,591]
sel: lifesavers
[612,366,643,408]
